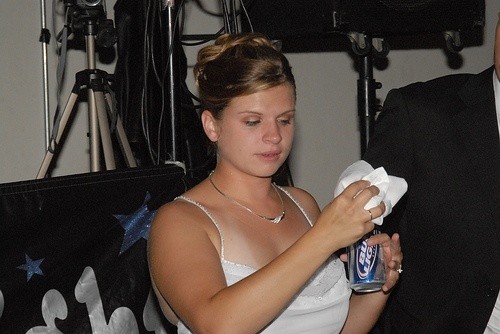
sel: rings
[389,263,403,273]
[369,210,373,220]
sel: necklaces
[209,169,286,224]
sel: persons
[366,14,500,334]
[147,33,405,334]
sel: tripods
[34,0,137,180]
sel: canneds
[345,226,387,293]
